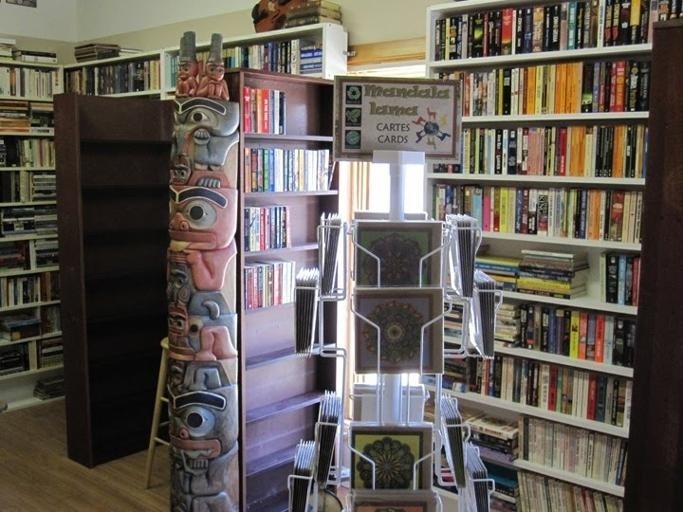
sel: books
[1,98,55,169]
[295,212,341,356]
[244,147,335,193]
[244,204,287,253]
[0,38,160,101]
[244,85,287,135]
[435,0,682,61]
[430,57,652,117]
[1,170,64,375]
[432,183,643,243]
[442,213,642,307]
[433,123,649,178]
[285,0,343,28]
[243,258,296,310]
[170,39,323,88]
[432,398,628,512]
[442,292,637,367]
[442,352,634,428]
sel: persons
[175,31,230,101]
